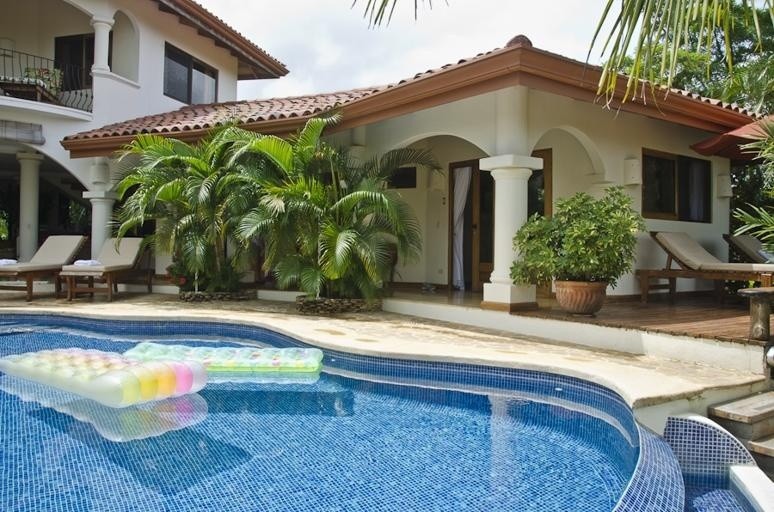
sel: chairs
[636,231,774,304]
[59,238,153,302]
[723,233,774,263]
[0,68,64,103]
[0,235,89,302]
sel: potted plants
[509,184,649,317]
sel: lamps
[624,159,641,186]
[717,174,733,198]
[0,38,15,56]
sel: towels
[74,260,101,266]
[0,259,17,265]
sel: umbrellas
[687,114,774,161]
